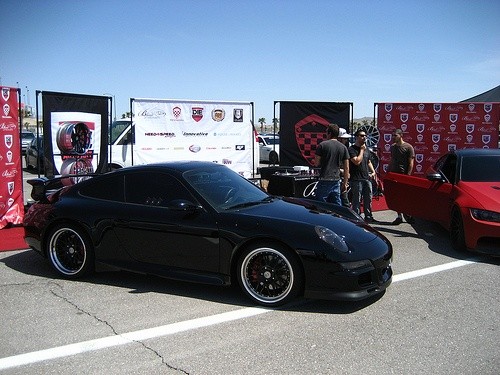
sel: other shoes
[393,218,402,225]
[364,217,380,224]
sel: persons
[348,130,380,224]
[315,123,350,205]
[386,129,415,225]
[337,127,351,207]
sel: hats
[338,128,351,138]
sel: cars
[20,131,45,172]
[255,131,280,164]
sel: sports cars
[384,148,500,254]
[23,161,393,306]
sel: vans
[106,118,133,168]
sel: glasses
[359,135,367,137]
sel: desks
[268,173,349,212]
